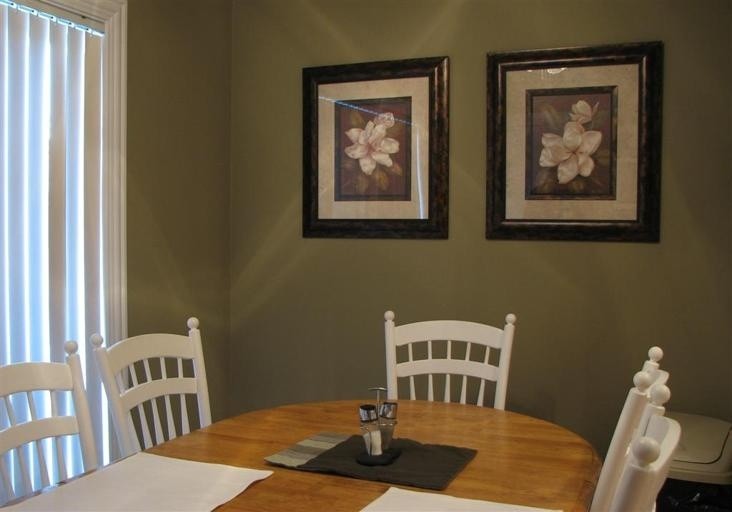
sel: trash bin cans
[655,411,732,512]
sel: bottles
[357,401,398,456]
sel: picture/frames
[301,53,451,242]
[485,40,666,246]
